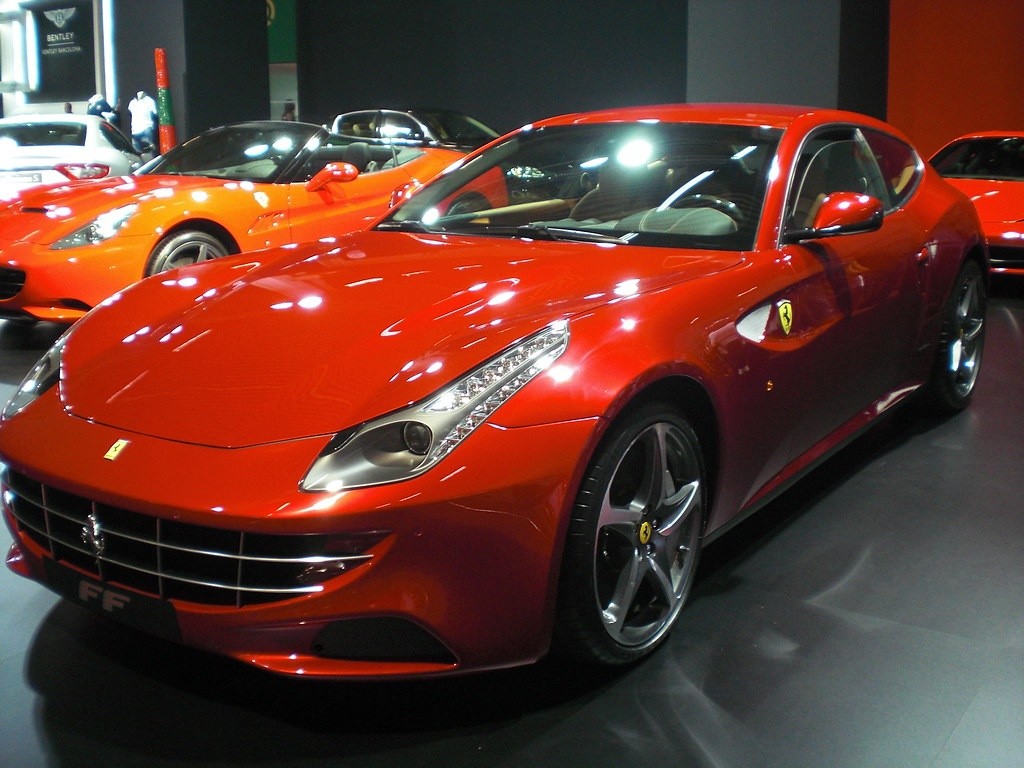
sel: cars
[0,102,991,680]
[0,115,155,203]
[321,107,501,153]
[926,131,1024,287]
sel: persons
[853,128,915,202]
[280,99,296,123]
[85,94,120,127]
[127,91,160,159]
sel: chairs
[334,142,379,175]
[568,153,652,228]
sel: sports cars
[0,111,511,328]
